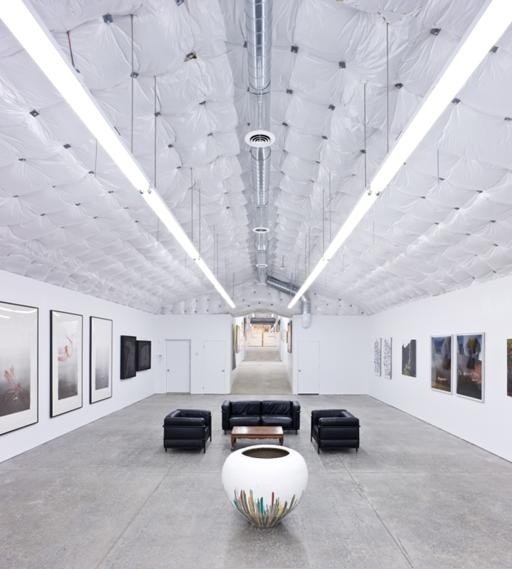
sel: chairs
[310,409,359,454]
[163,408,212,453]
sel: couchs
[221,399,301,435]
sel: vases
[221,444,310,528]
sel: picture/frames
[0,301,151,437]
[372,331,512,405]
